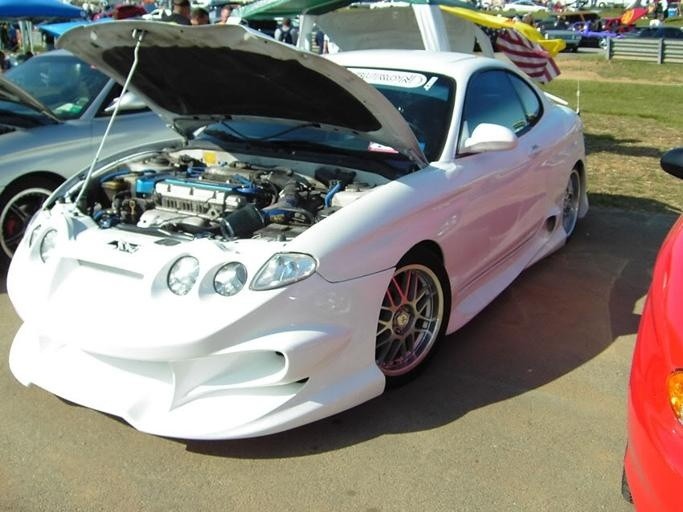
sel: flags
[474,23,561,86]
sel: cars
[498,0,551,12]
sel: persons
[314,31,325,55]
[189,7,210,26]
[219,4,234,24]
[273,17,299,47]
[321,33,340,54]
[161,1,192,26]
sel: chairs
[12,64,103,117]
